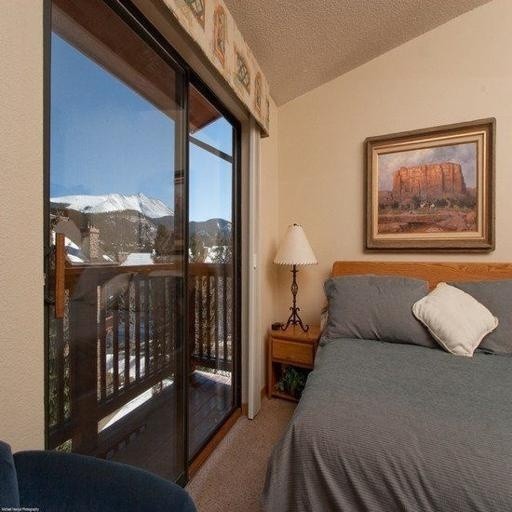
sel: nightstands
[262,325,322,406]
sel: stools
[0,438,196,512]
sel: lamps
[271,221,316,332]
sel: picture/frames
[362,116,497,256]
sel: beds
[258,255,512,511]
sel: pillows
[441,279,510,358]
[406,277,500,359]
[319,271,434,350]
